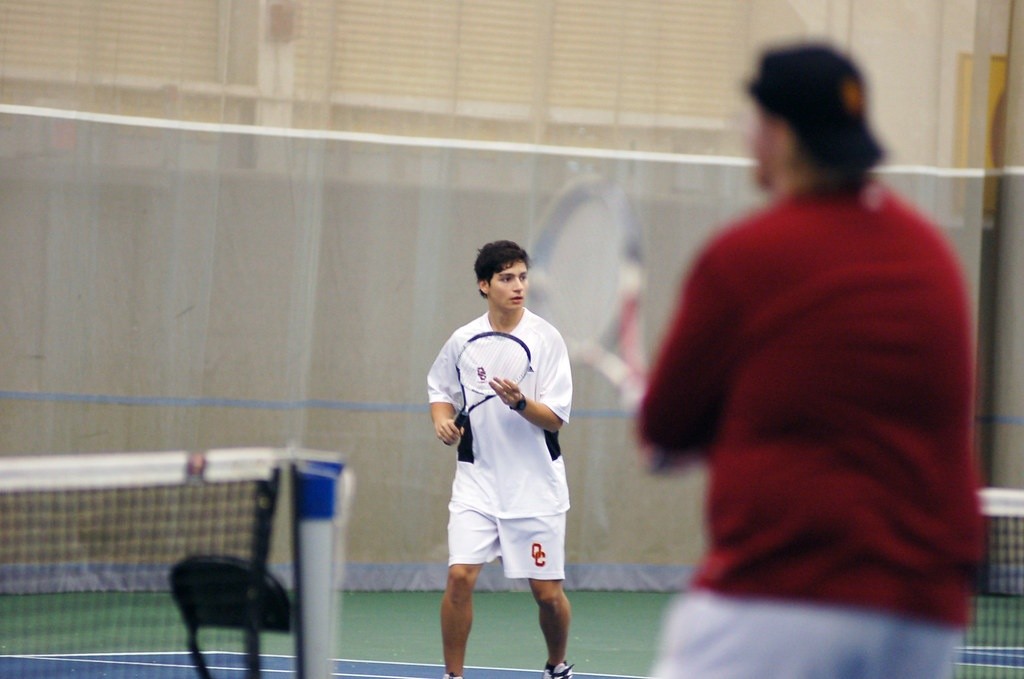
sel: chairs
[168,555,290,678]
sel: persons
[425,240,573,679]
[632,43,991,679]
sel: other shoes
[543,659,575,679]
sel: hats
[750,48,882,170]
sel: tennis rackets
[444,332,530,445]
